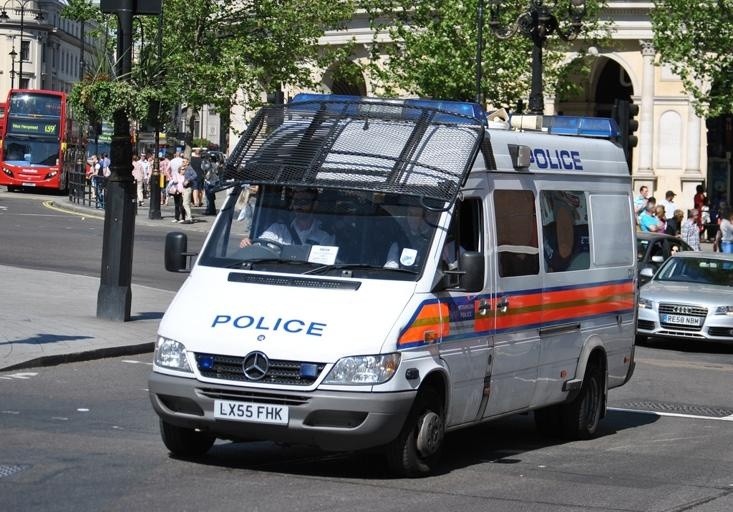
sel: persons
[633,184,733,268]
[679,261,709,282]
[87,148,259,233]
[382,202,468,270]
[239,187,336,250]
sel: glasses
[291,198,310,206]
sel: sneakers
[172,219,194,224]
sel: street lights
[6,45,17,88]
[487,0,590,113]
[0,0,45,88]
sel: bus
[84,115,139,181]
[0,87,90,195]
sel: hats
[666,190,677,196]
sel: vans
[147,91,646,478]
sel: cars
[633,227,696,289]
[637,250,733,347]
